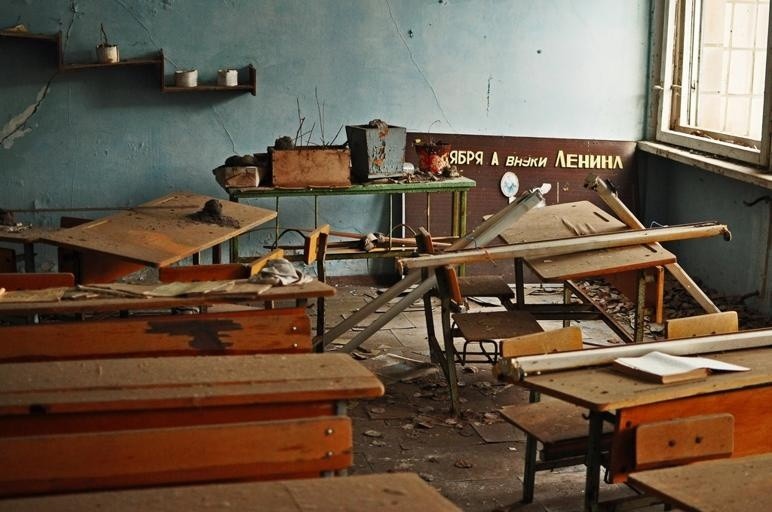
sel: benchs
[2,308,337,439]
[2,416,355,500]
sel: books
[612,351,754,384]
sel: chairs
[433,263,545,416]
[415,227,516,309]
[244,228,329,276]
[634,412,736,472]
[0,270,78,321]
[664,309,740,343]
[498,326,618,506]
[158,263,247,314]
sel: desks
[498,346,770,509]
[1,272,336,309]
[230,175,476,276]
[628,452,770,509]
[482,198,679,344]
[2,352,386,476]
[37,188,278,278]
[3,471,465,511]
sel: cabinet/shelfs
[0,29,257,94]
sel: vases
[175,65,237,87]
[96,43,119,61]
[213,120,451,189]
